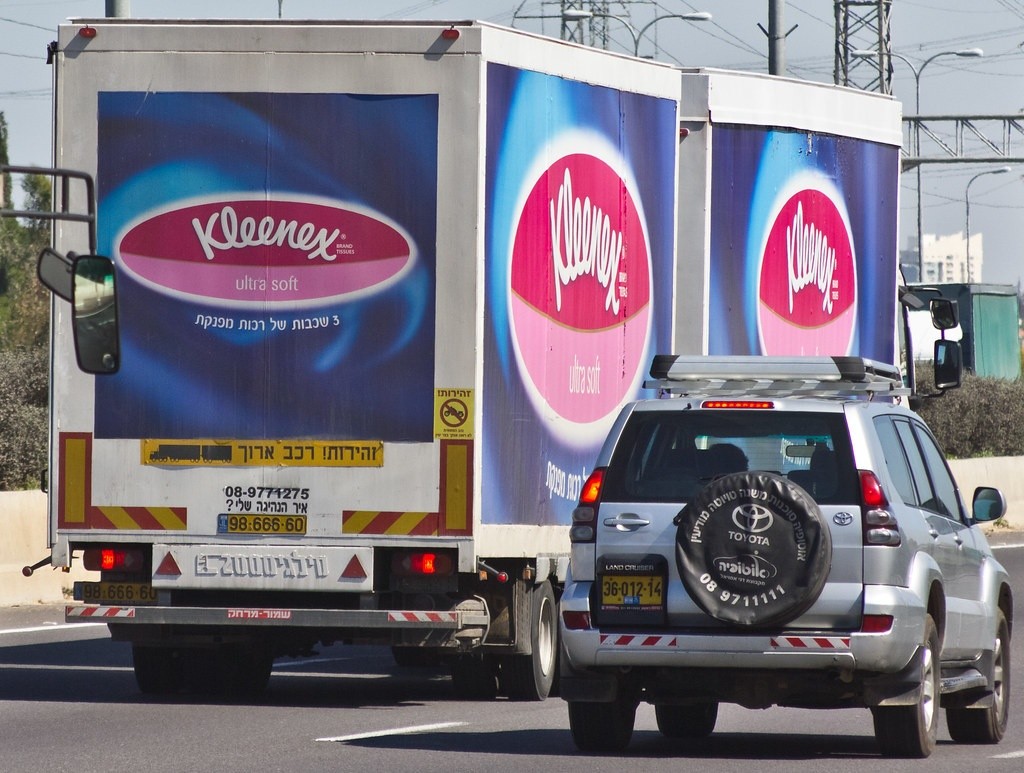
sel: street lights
[849,43,984,284]
[964,166,1011,284]
[563,8,713,58]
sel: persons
[704,443,748,481]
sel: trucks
[21,15,966,701]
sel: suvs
[554,351,1016,761]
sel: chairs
[790,445,860,505]
[635,444,749,498]
